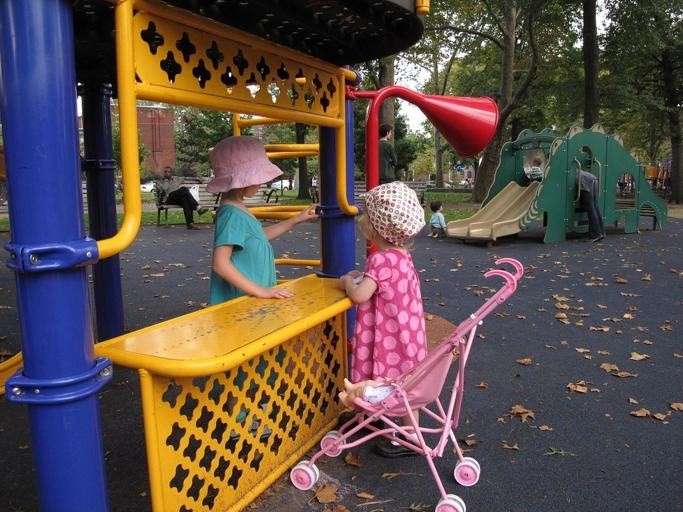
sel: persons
[529,159,544,178]
[378,124,399,186]
[577,168,608,242]
[338,180,428,458]
[205,135,319,443]
[427,200,446,238]
[157,166,209,230]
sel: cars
[529,166,543,181]
[270,180,294,192]
[140,180,156,193]
[443,179,474,190]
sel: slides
[446,181,541,243]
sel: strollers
[284,257,524,511]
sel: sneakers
[592,233,606,242]
[338,411,384,428]
[187,224,201,230]
[375,433,422,457]
[198,208,208,214]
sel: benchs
[615,193,668,230]
[308,179,428,212]
[149,182,278,228]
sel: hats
[365,181,426,246]
[205,135,284,193]
